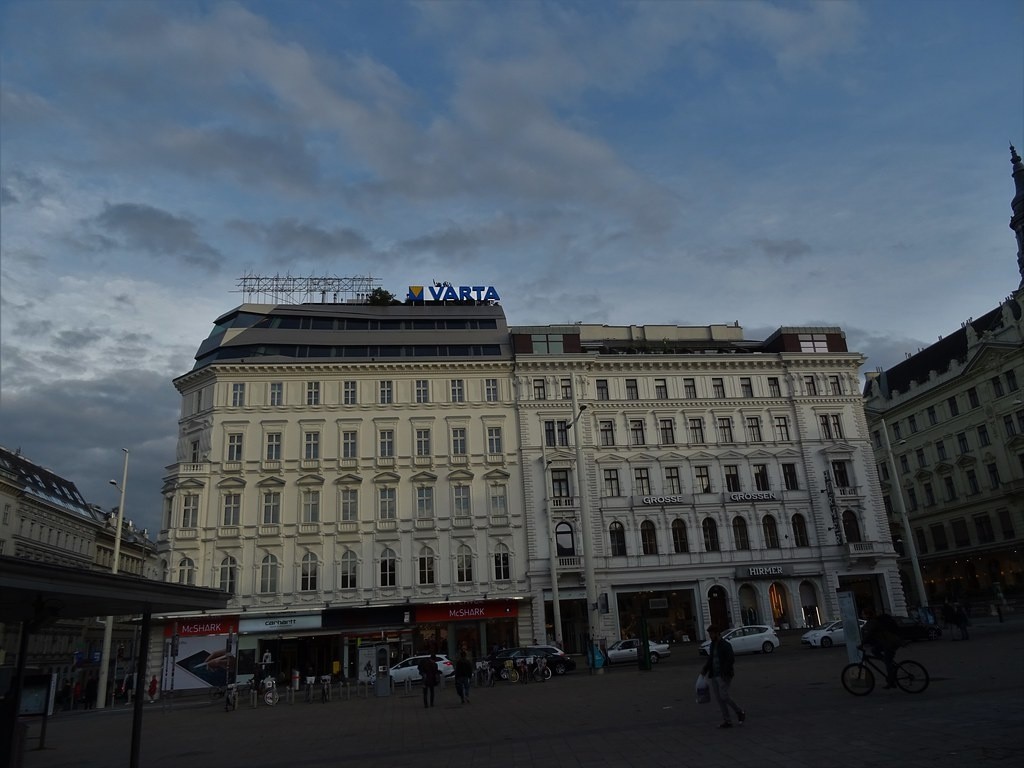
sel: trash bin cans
[292,671,301,691]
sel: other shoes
[717,719,734,728]
[737,711,745,725]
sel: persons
[445,651,472,704]
[858,608,904,689]
[147,675,157,700]
[419,654,443,708]
[700,625,746,728]
[941,600,970,641]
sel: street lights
[96,448,130,709]
[543,459,564,650]
[880,418,929,607]
[565,405,604,675]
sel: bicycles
[478,654,552,687]
[208,676,280,706]
[841,644,929,696]
[304,673,334,704]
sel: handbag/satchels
[696,674,711,703]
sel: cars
[476,645,577,681]
[862,616,942,641]
[370,654,456,689]
[699,625,781,657]
[603,639,671,665]
[800,619,867,648]
